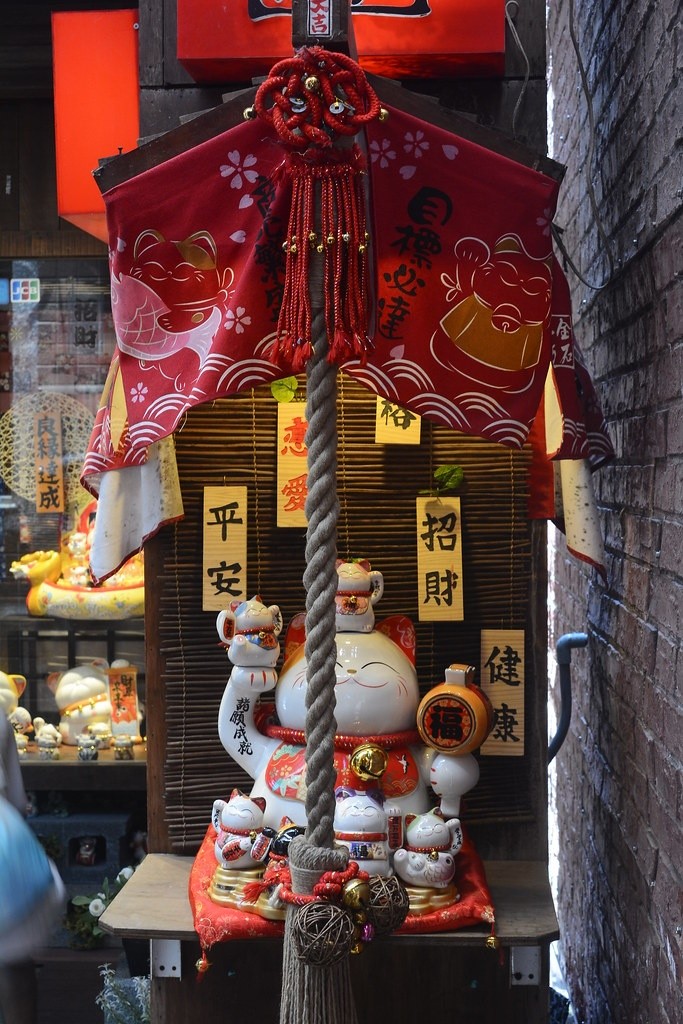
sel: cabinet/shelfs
[1,613,149,793]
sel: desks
[97,853,561,1024]
